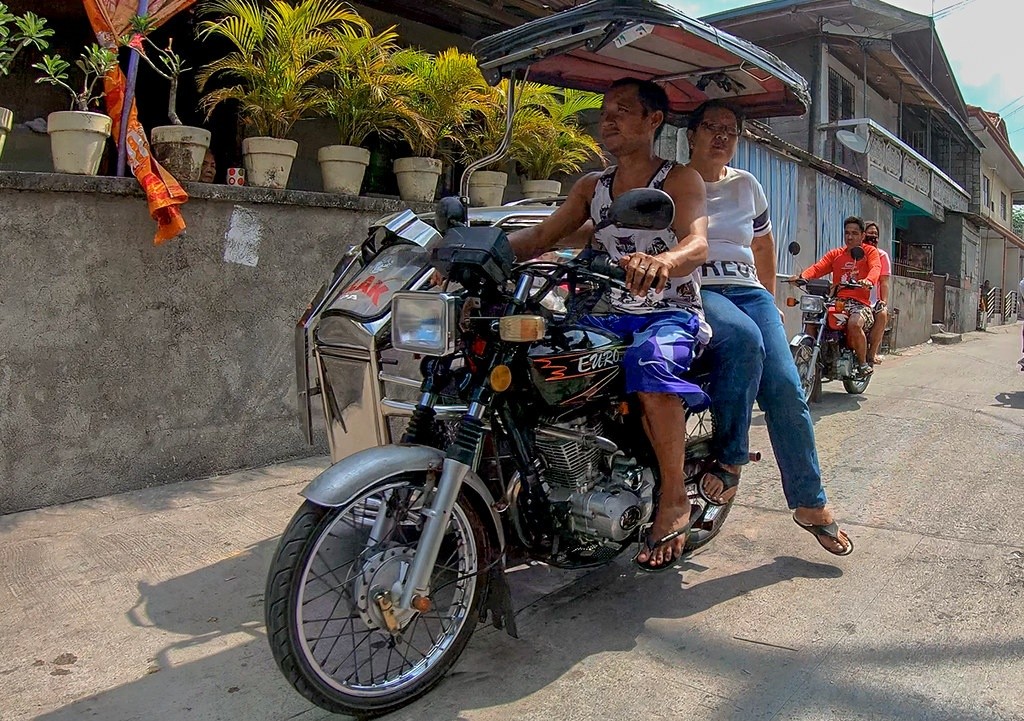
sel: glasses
[700,121,741,135]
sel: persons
[429,76,712,574]
[863,221,891,365]
[788,216,882,376]
[198,148,216,183]
[682,98,853,557]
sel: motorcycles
[265,0,813,721]
[780,241,875,406]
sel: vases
[227,165,245,187]
[150,125,211,180]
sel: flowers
[130,15,183,125]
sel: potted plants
[366,38,489,200]
[190,1,343,187]
[429,77,546,204]
[40,14,126,173]
[0,4,52,162]
[306,13,423,192]
[499,83,607,200]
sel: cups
[227,168,246,187]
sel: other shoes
[868,356,882,364]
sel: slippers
[792,512,853,555]
[698,462,742,506]
[857,363,874,376]
[637,504,701,573]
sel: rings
[638,266,647,270]
